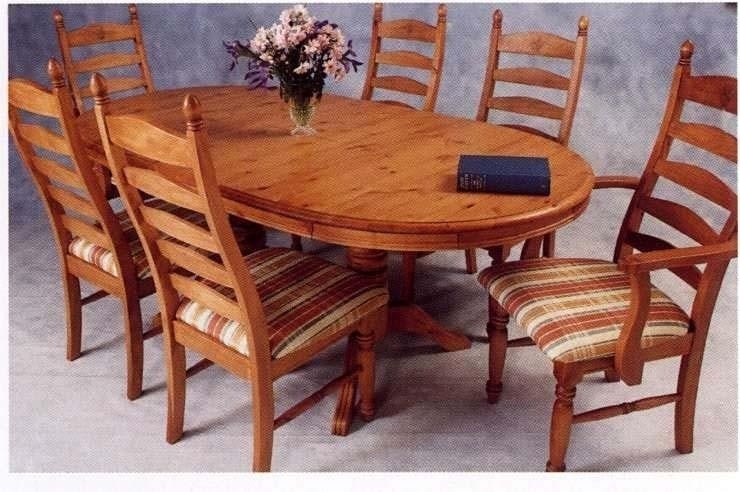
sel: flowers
[219,1,361,121]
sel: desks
[75,81,601,425]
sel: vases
[286,78,318,137]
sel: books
[456,154,551,196]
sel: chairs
[46,4,273,254]
[10,53,256,407]
[480,33,736,471]
[291,4,452,304]
[87,64,391,472]
[450,6,589,286]
